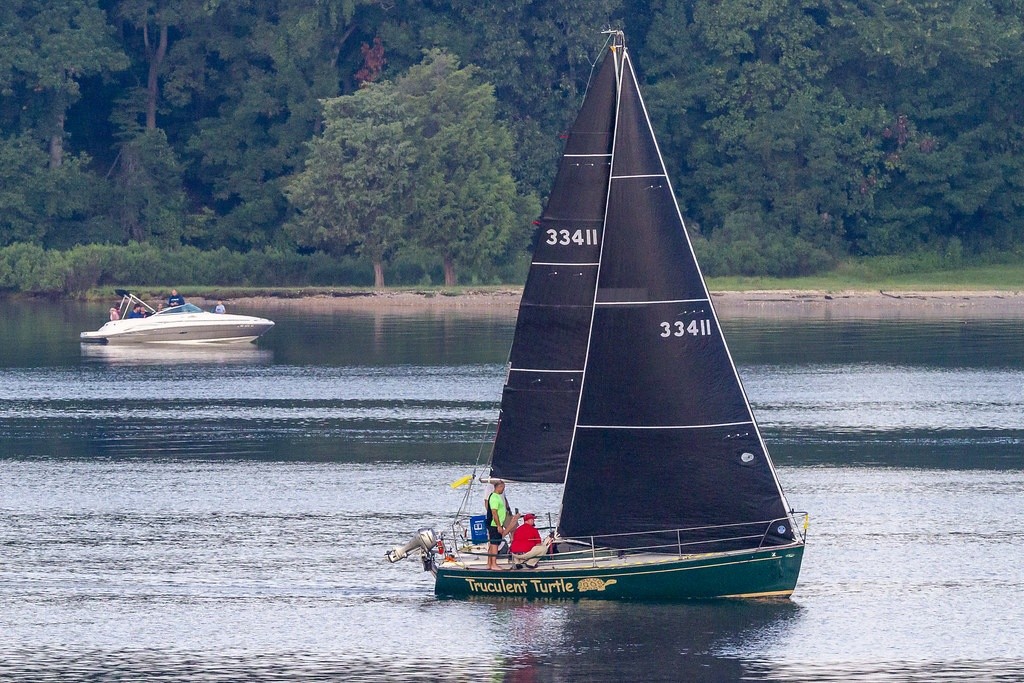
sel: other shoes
[525,562,539,569]
[515,563,523,569]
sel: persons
[486,481,506,570]
[110,288,184,320]
[484,483,513,518]
[509,514,548,570]
[215,299,226,314]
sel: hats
[523,514,537,521]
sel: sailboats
[383,23,809,602]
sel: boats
[79,288,275,345]
